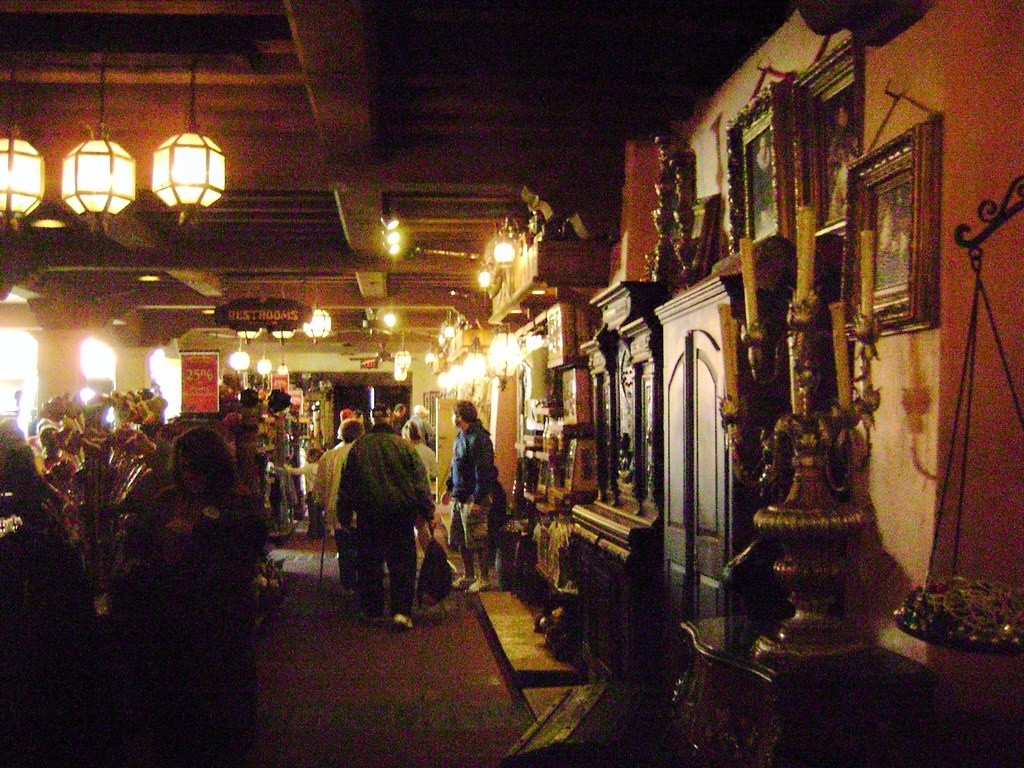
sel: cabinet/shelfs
[572,274,746,681]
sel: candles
[860,230,879,318]
[718,299,740,410]
[790,204,815,302]
[741,237,761,333]
[828,301,853,408]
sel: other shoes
[394,614,414,629]
[359,610,383,624]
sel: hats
[267,389,292,414]
[240,389,259,404]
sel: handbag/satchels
[417,536,454,609]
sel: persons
[1,387,507,768]
[337,402,440,629]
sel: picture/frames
[726,29,859,254]
[841,116,944,340]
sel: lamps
[393,334,412,387]
[237,328,264,344]
[229,338,251,374]
[270,329,296,346]
[303,308,333,346]
[152,55,226,232]
[487,324,522,390]
[493,242,516,269]
[257,356,272,379]
[61,51,136,231]
[0,48,45,230]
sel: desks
[680,617,936,768]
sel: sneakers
[465,577,491,593]
[451,576,478,589]
